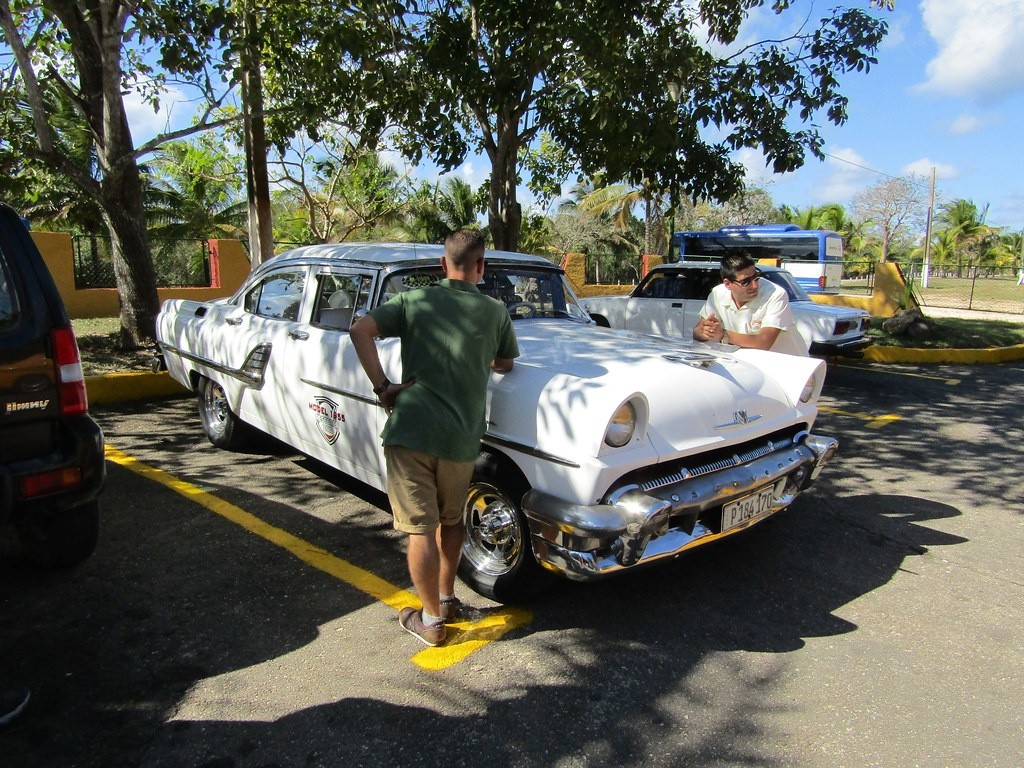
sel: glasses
[732,266,762,286]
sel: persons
[693,252,810,360]
[349,227,521,652]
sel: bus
[672,224,843,295]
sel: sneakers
[440,596,464,623]
[398,597,446,647]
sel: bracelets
[373,379,390,394]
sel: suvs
[0,199,109,573]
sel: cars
[577,260,875,360]
[155,242,839,603]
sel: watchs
[720,328,731,345]
